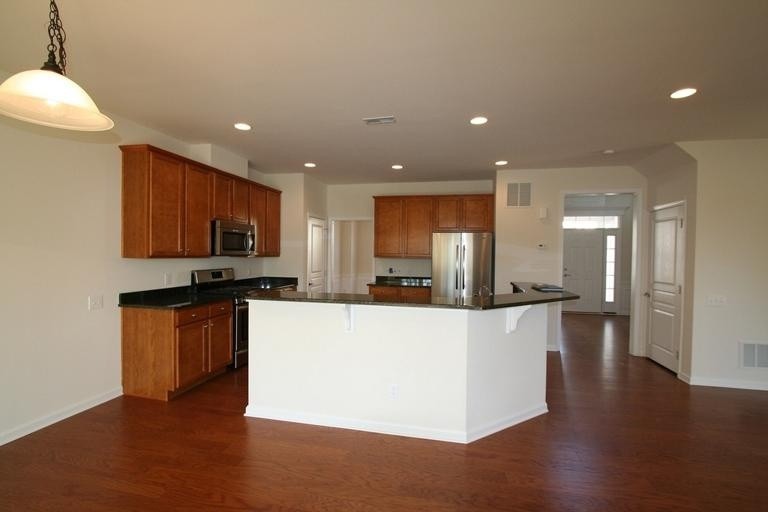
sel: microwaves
[212,220,256,257]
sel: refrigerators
[431,232,492,298]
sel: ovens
[233,295,248,368]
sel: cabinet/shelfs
[118,144,283,258]
[120,300,233,402]
[372,194,494,257]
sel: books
[531,284,565,293]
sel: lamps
[0,0,115,132]
